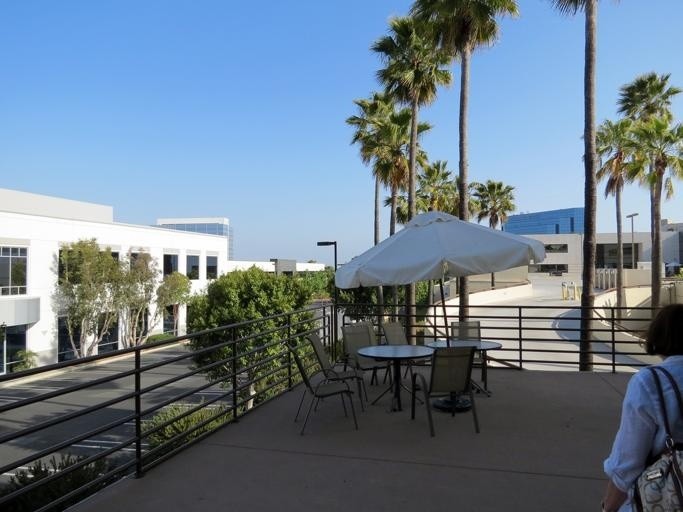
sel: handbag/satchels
[634,447,683,512]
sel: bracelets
[601,500,606,510]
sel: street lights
[316,242,338,343]
[627,213,639,269]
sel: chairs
[285,320,488,439]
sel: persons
[599,302,683,512]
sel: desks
[429,338,503,398]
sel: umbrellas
[334,210,547,411]
[666,261,682,268]
